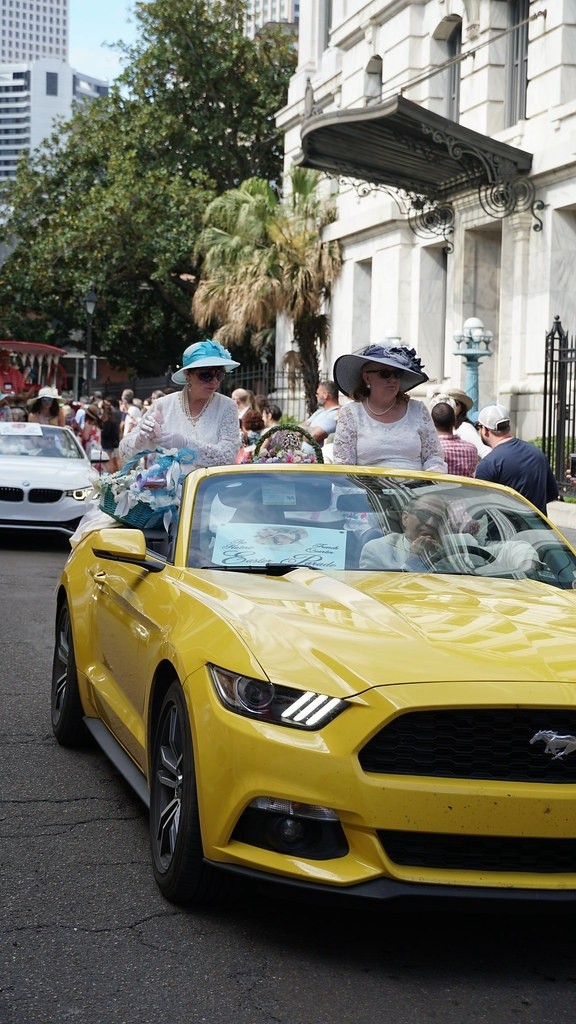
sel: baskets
[96,449,183,529]
[215,425,330,512]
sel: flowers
[241,448,317,465]
[208,338,233,361]
[108,467,181,512]
[384,344,425,373]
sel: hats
[83,405,100,420]
[442,388,474,411]
[26,387,62,407]
[171,339,241,385]
[478,405,511,432]
[333,343,430,399]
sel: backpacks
[101,401,116,424]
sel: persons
[359,493,550,576]
[333,345,448,473]
[430,389,493,477]
[119,340,240,476]
[232,388,282,446]
[0,387,174,474]
[298,381,341,454]
[475,405,559,517]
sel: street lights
[453,317,495,422]
[83,279,100,396]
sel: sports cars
[0,421,103,537]
[50,463,576,904]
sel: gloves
[137,415,157,445]
[157,431,189,450]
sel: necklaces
[184,389,210,419]
[367,396,397,415]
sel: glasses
[188,367,228,383]
[366,368,405,379]
[40,397,53,403]
[476,422,484,430]
[454,399,461,406]
[407,508,446,525]
